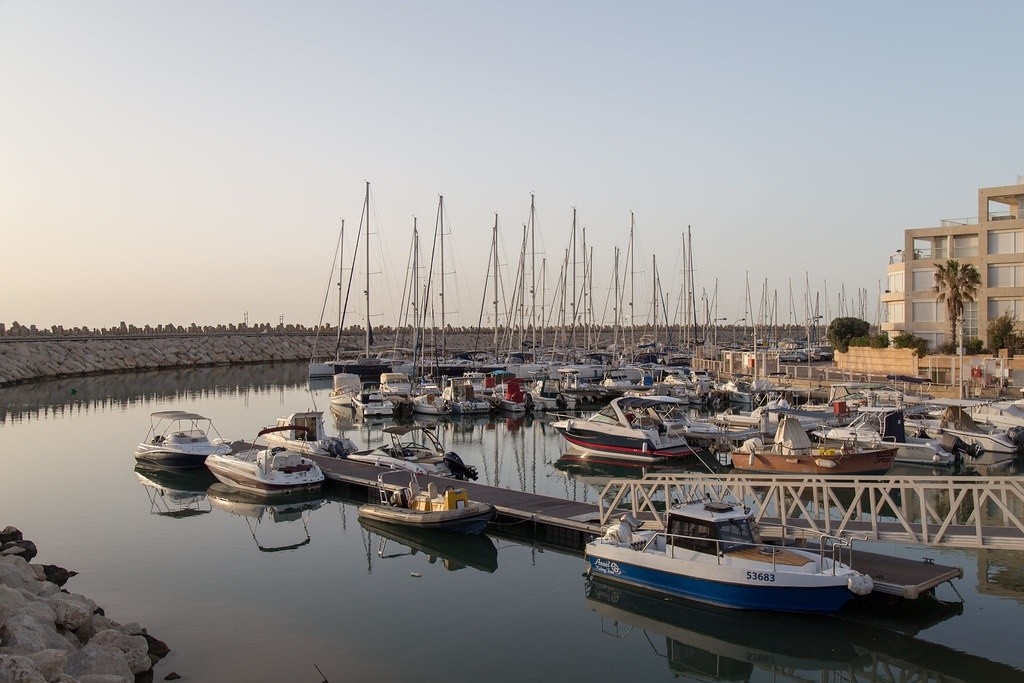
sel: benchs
[754,445,773,454]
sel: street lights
[733,318,747,352]
[712,318,729,361]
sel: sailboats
[310,182,1023,485]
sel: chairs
[410,480,444,512]
[269,452,298,469]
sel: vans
[802,349,821,362]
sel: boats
[359,468,501,533]
[204,481,325,557]
[582,497,875,619]
[355,518,499,576]
[583,584,867,683]
[134,466,213,516]
[203,425,325,500]
[134,411,233,465]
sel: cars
[817,349,834,361]
[779,351,809,363]
